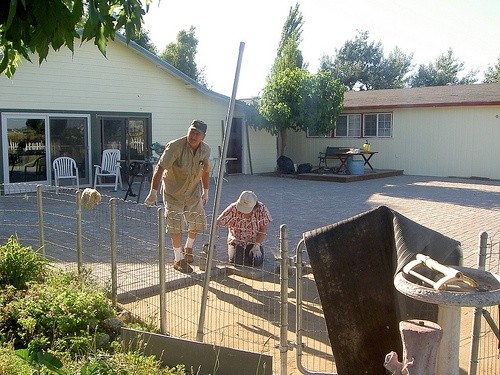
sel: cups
[354,149,360,153]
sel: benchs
[318,146,353,173]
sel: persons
[215,191,273,266]
[144,120,211,273]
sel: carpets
[302,205,463,375]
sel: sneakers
[183,248,194,263]
[174,259,193,273]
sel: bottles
[363,140,370,151]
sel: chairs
[8,153,47,182]
[53,148,124,192]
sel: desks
[336,151,379,175]
[117,159,158,205]
[209,158,238,185]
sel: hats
[189,120,207,134]
[236,191,257,214]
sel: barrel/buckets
[346,160,364,175]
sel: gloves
[249,242,263,266]
[202,189,208,206]
[144,189,157,208]
